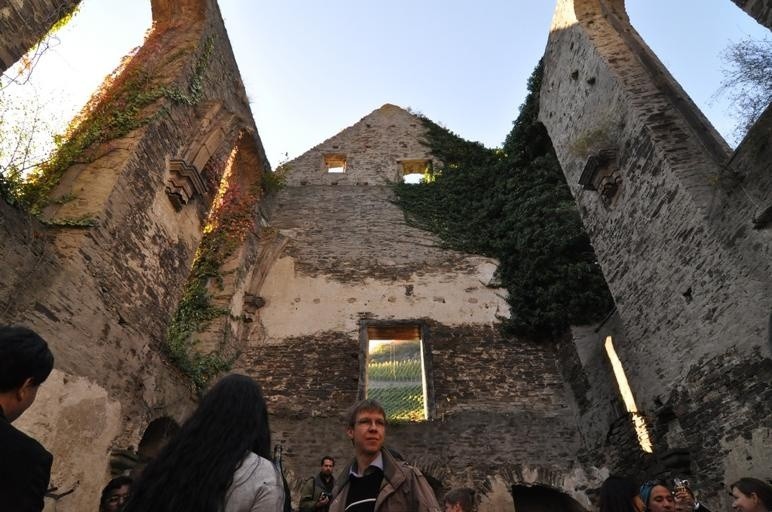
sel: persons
[0,323,57,511]
[298,456,336,511]
[443,486,476,511]
[645,478,712,512]
[97,369,287,511]
[596,474,649,511]
[730,477,772,512]
[329,398,442,511]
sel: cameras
[320,491,333,502]
